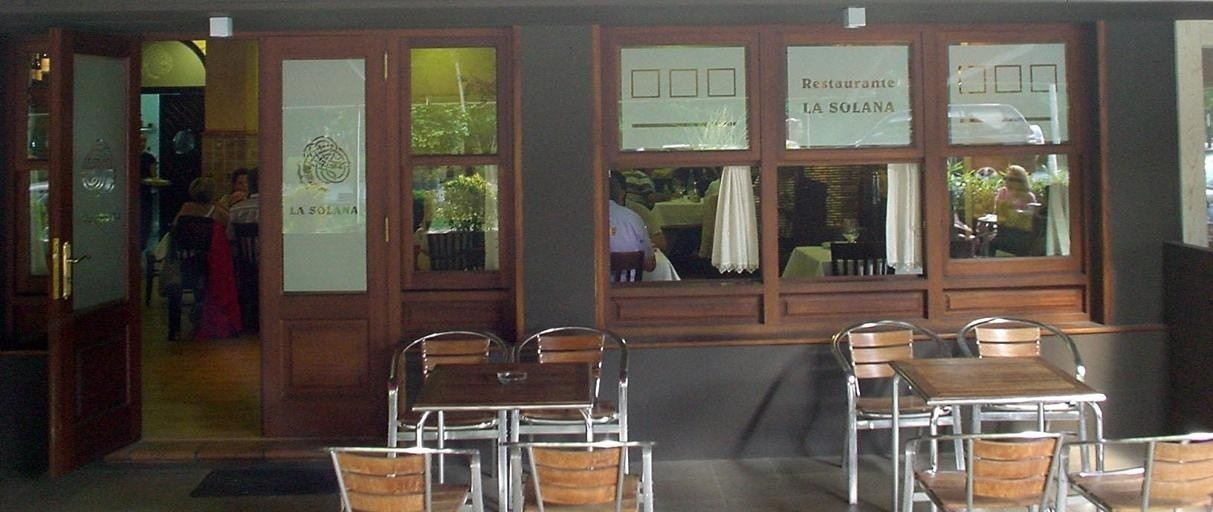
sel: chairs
[229,218,260,323]
[166,215,230,343]
[328,442,486,511]
[828,323,967,506]
[412,169,1050,279]
[957,317,1089,475]
[497,439,655,511]
[900,421,1056,512]
[512,323,631,477]
[385,327,508,496]
[1056,414,1212,512]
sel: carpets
[187,465,337,498]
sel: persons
[1015,185,1049,256]
[138,134,160,253]
[993,165,1057,216]
[170,167,260,308]
[608,166,725,283]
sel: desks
[408,363,594,511]
[885,357,1108,512]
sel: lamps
[204,12,233,40]
[844,1,867,33]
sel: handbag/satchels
[157,243,185,301]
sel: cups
[842,219,860,242]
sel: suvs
[852,103,1044,179]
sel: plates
[498,371,526,384]
